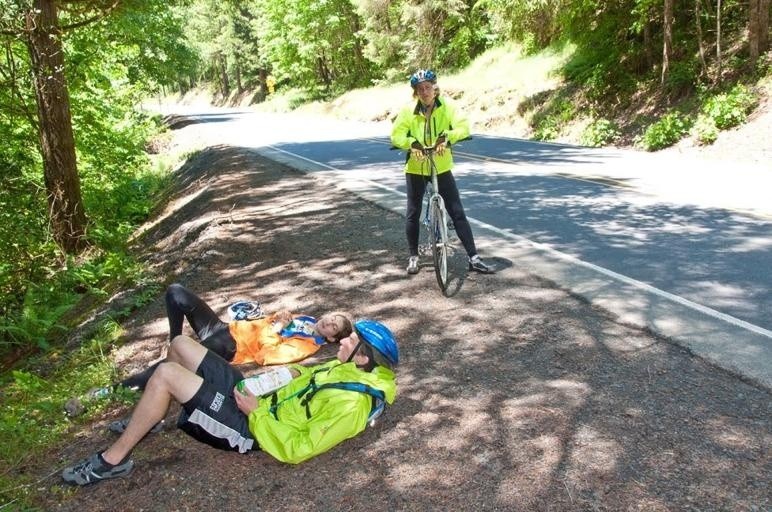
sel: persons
[62,318,398,485]
[90,282,352,397]
[389,69,497,276]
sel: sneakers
[406,254,421,273]
[64,386,164,433]
[62,450,134,486]
[468,257,495,273]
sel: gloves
[435,136,447,156]
[411,141,427,161]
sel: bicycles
[389,136,473,291]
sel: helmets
[227,300,266,321]
[353,319,399,365]
[411,70,437,87]
